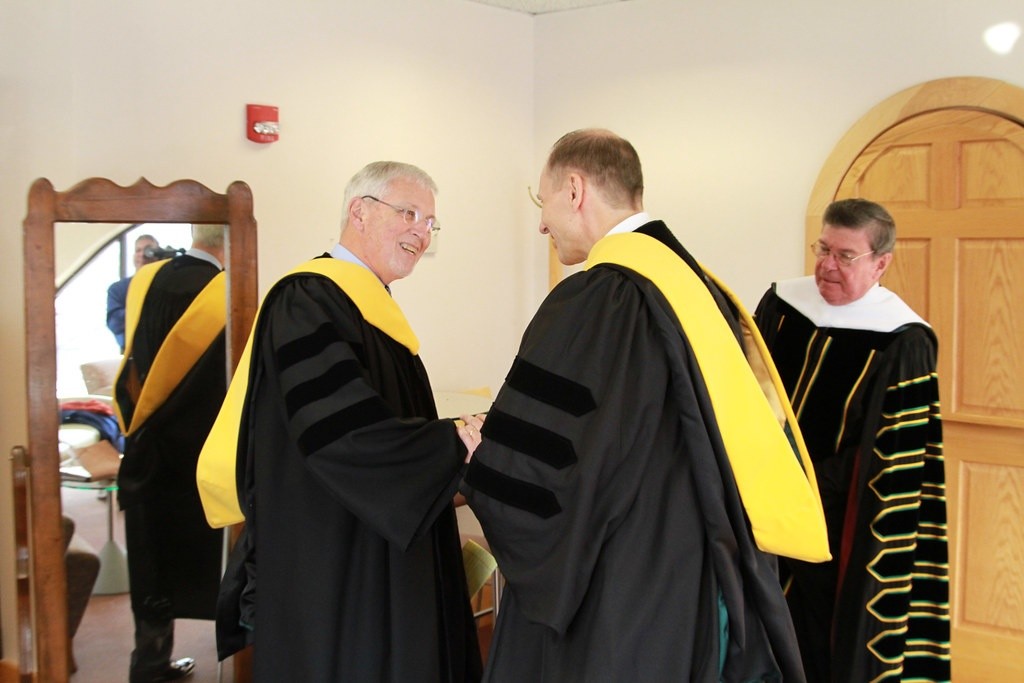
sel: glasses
[362,195,440,237]
[811,240,875,266]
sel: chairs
[463,540,497,604]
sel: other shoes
[170,656,196,677]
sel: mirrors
[8,176,259,683]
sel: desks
[59,479,119,542]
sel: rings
[470,429,478,436]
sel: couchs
[54,354,226,596]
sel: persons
[459,130,832,683]
[106,235,159,354]
[753,198,953,683]
[195,162,469,683]
[113,224,226,683]
